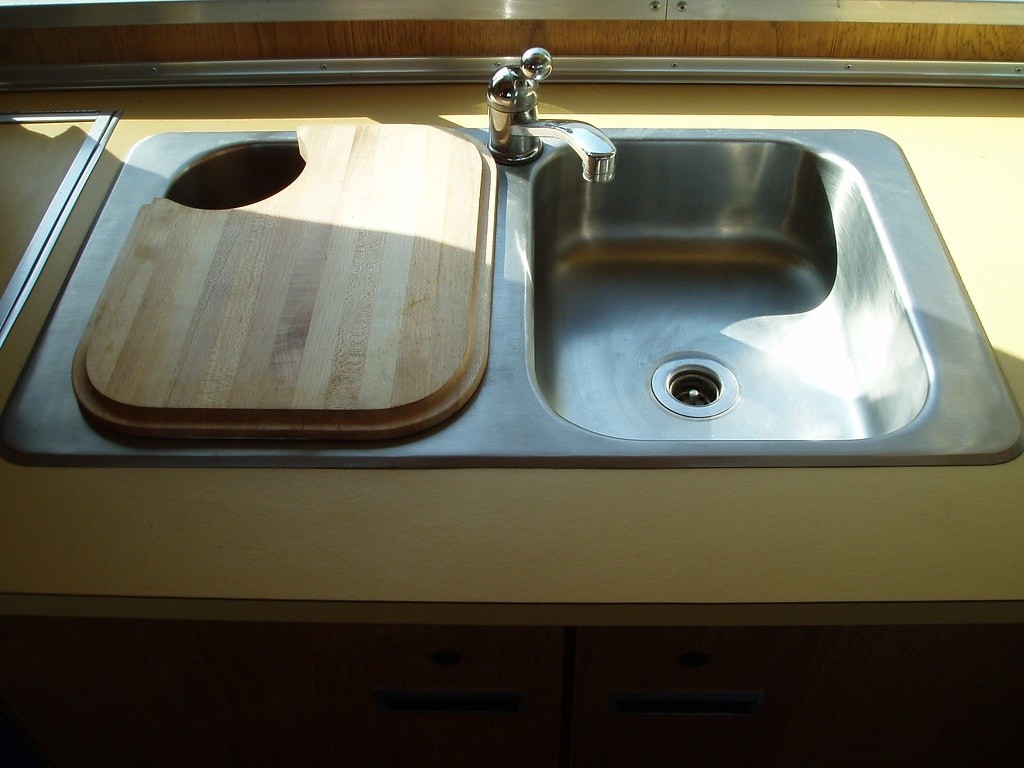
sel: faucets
[485,46,620,186]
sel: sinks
[1,129,537,468]
[491,124,1024,470]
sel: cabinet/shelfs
[0,601,1024,768]
